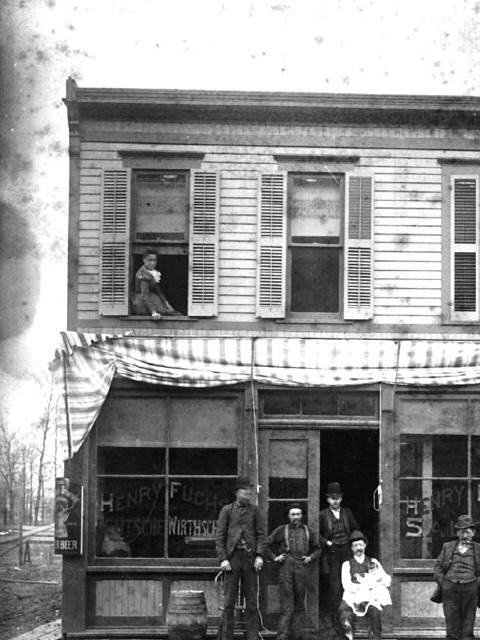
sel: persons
[134,250,184,322]
[432,510,480,640]
[102,525,130,558]
[217,481,390,640]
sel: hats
[324,482,344,498]
[453,514,476,529]
[285,502,304,512]
[347,530,368,544]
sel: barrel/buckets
[167,589,208,640]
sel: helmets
[231,476,254,491]
